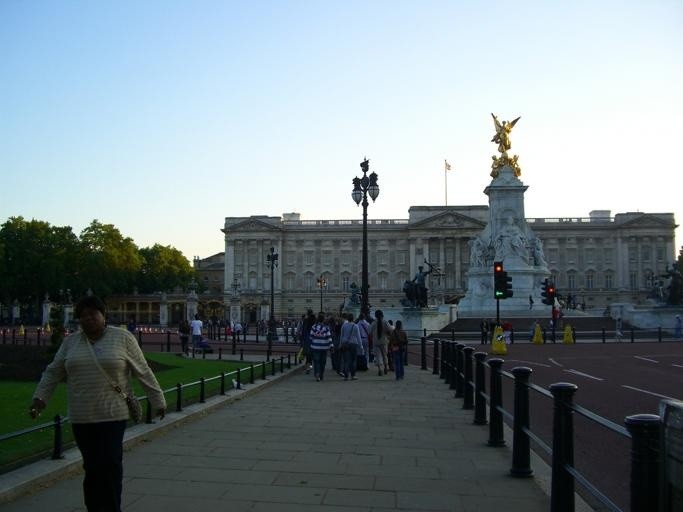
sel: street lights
[266,247,279,340]
[351,157,380,313]
[317,276,327,313]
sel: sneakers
[315,369,388,382]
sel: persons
[176,308,410,383]
[27,297,166,511]
[559,293,585,312]
[467,216,548,268]
[496,120,511,153]
[411,265,432,308]
[552,307,559,327]
[558,310,564,330]
[489,155,498,178]
[126,317,135,331]
[528,319,542,340]
[528,294,533,311]
[479,318,512,344]
[349,282,359,304]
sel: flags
[445,163,451,170]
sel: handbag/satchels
[340,343,350,352]
[127,395,143,425]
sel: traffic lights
[541,278,554,305]
[494,262,513,300]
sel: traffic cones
[18,325,24,335]
[532,323,544,345]
[492,325,506,355]
[563,325,573,344]
[46,324,51,332]
